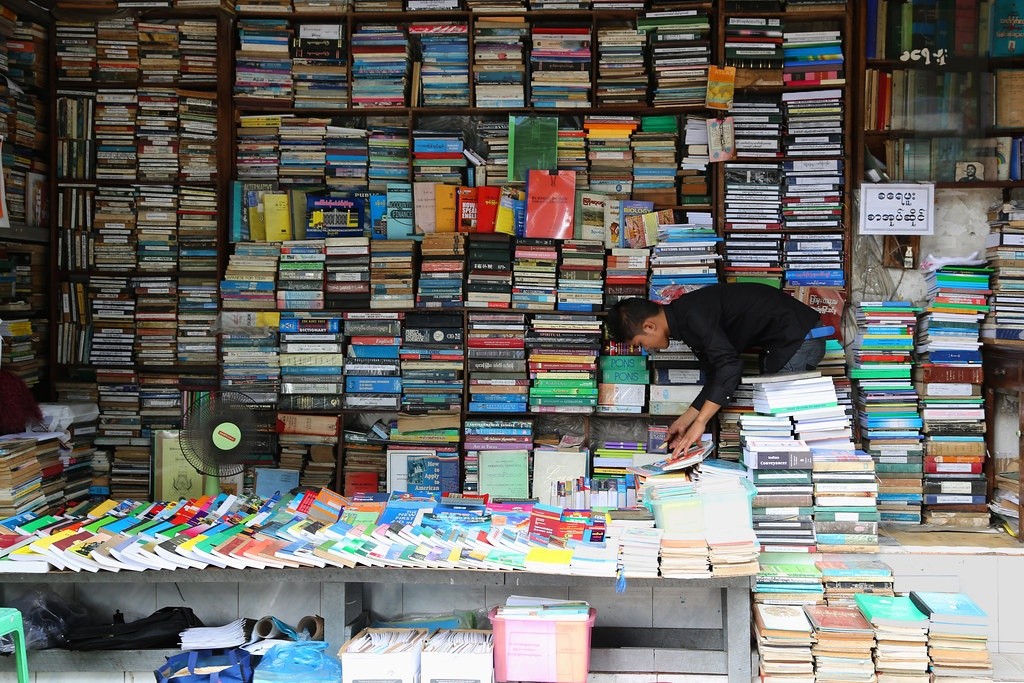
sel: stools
[0,608,30,683]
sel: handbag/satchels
[67,605,205,651]
[252,616,342,683]
[153,648,252,683]
[0,583,90,656]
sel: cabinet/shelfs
[237,0,718,503]
[0,0,58,398]
[48,0,231,499]
[853,0,1023,189]
[718,0,852,305]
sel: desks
[982,342,1024,542]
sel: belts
[803,326,835,340]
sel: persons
[603,280,830,458]
[958,164,984,183]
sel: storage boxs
[420,628,495,683]
[486,605,598,683]
[337,626,429,683]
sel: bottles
[904,246,913,268]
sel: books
[0,0,1024,683]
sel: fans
[178,389,270,497]
